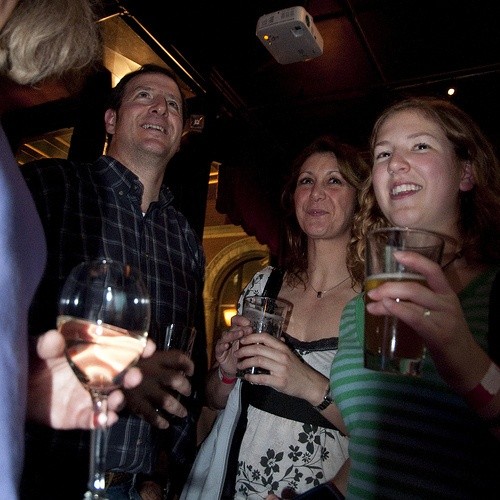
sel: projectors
[256,6,324,65]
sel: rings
[423,309,431,319]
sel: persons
[265,98,500,500]
[20,63,201,500]
[0,1,157,500]
[179,133,376,499]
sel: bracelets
[314,388,333,411]
[218,369,237,384]
[466,363,499,412]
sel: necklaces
[301,274,352,299]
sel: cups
[164,323,198,400]
[235,295,293,376]
[363,227,444,378]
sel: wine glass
[59,259,150,500]
